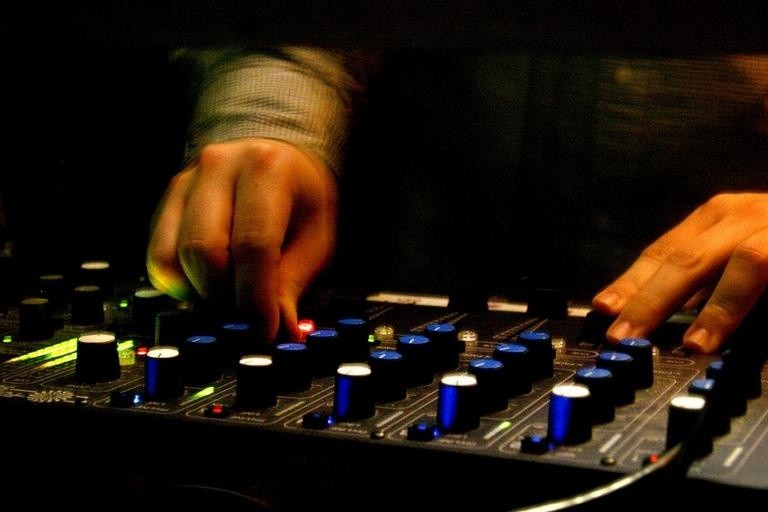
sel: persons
[143,35,768,355]
